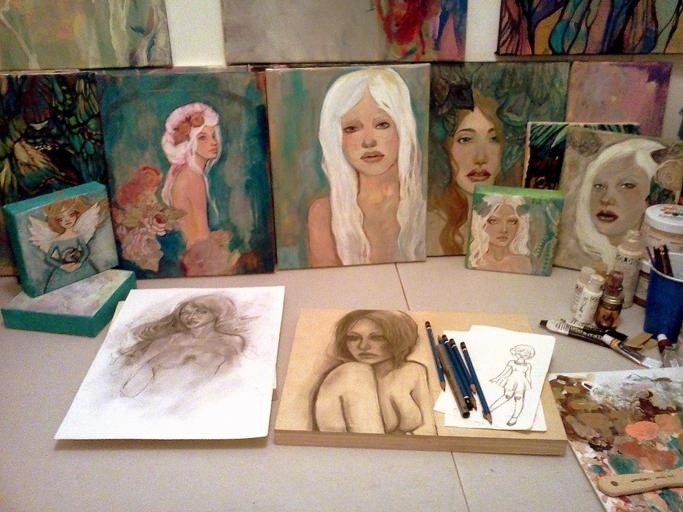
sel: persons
[306,67,426,267]
[470,196,535,272]
[427,81,506,257]
[108,295,261,400]
[161,101,222,248]
[314,310,437,436]
[574,139,667,270]
[28,200,105,293]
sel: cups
[644,252,682,343]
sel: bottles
[569,228,653,333]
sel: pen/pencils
[425,321,492,424]
[646,244,673,277]
